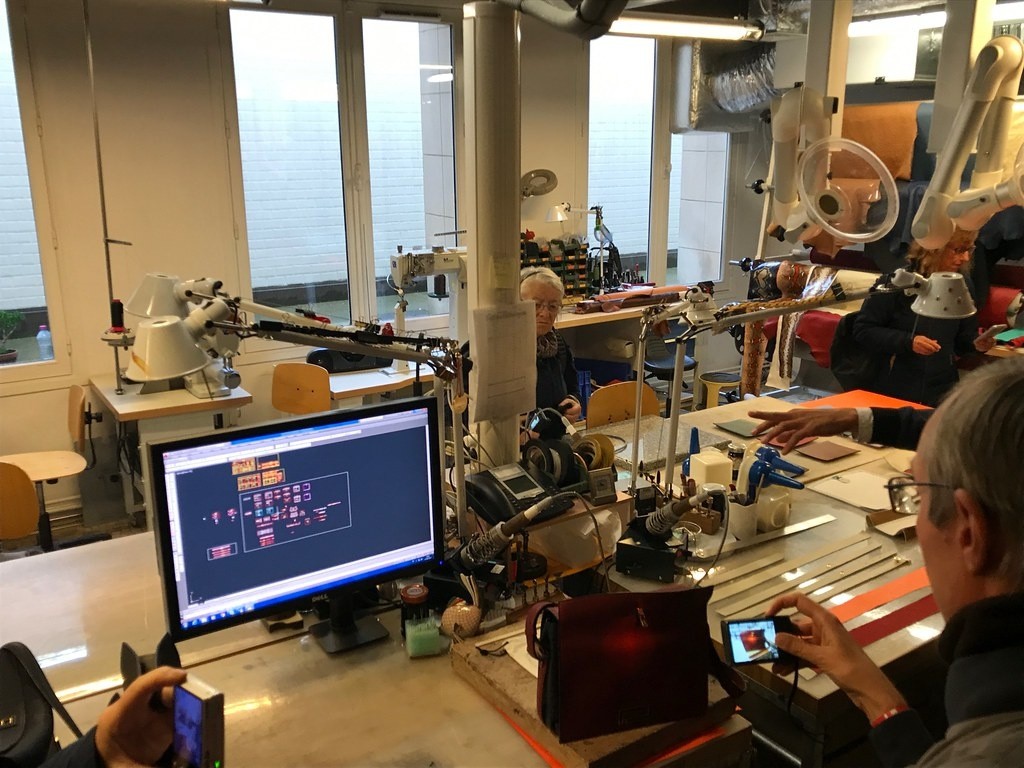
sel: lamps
[124,267,469,549]
[606,9,763,41]
[545,202,604,295]
[623,267,978,501]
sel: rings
[991,342,996,347]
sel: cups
[727,491,762,539]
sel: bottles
[399,584,431,640]
[37,325,53,360]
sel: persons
[37,666,188,768]
[860,223,997,408]
[748,406,937,456]
[461,266,582,452]
[765,354,1024,768]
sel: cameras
[721,615,798,665]
[172,675,225,768]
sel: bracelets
[870,706,911,728]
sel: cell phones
[973,324,1009,343]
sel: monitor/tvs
[146,395,445,654]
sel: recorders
[307,347,394,373]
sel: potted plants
[0,311,25,364]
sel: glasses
[884,476,960,515]
[947,245,977,254]
[533,298,565,313]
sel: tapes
[573,433,615,470]
[545,440,574,487]
[523,438,554,474]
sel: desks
[89,372,253,526]
[553,300,702,411]
[0,395,945,768]
[329,361,433,409]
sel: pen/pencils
[622,272,625,282]
[635,264,638,275]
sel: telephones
[465,459,574,530]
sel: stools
[694,373,742,411]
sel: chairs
[586,381,660,430]
[644,335,696,418]
[0,385,103,559]
[272,363,331,418]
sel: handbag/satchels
[524,585,717,744]
